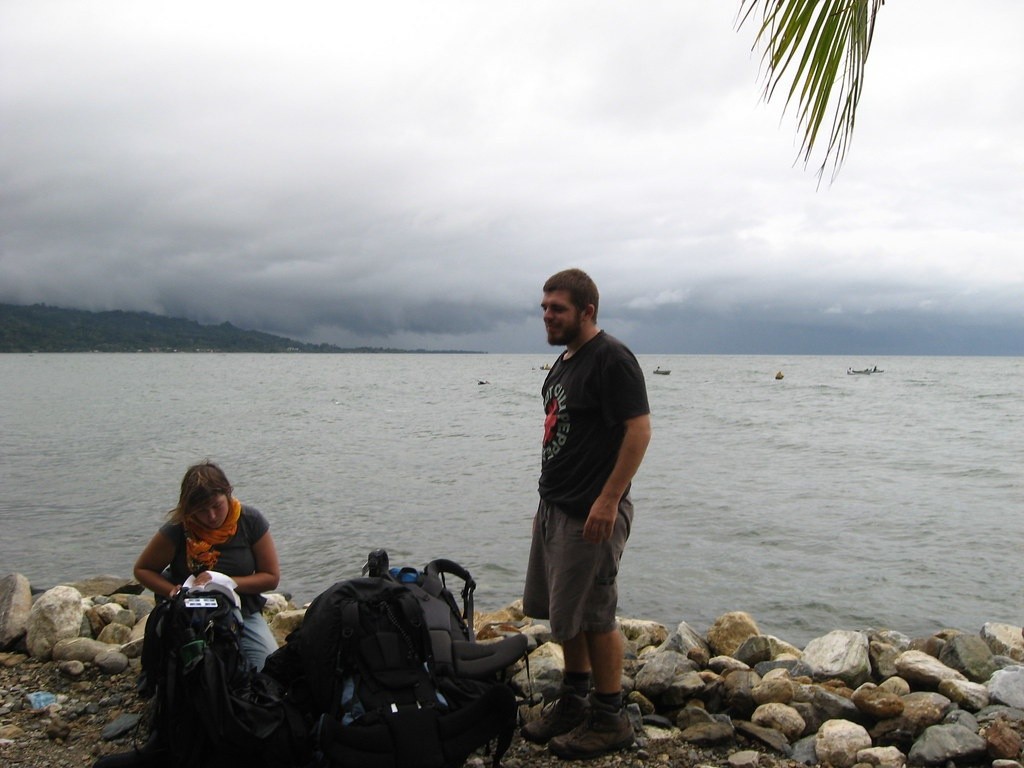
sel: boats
[653,365,672,376]
[847,365,882,376]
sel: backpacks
[139,548,532,768]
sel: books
[182,571,242,610]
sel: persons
[522,269,650,758]
[133,463,280,673]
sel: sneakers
[521,694,592,742]
[548,695,635,760]
